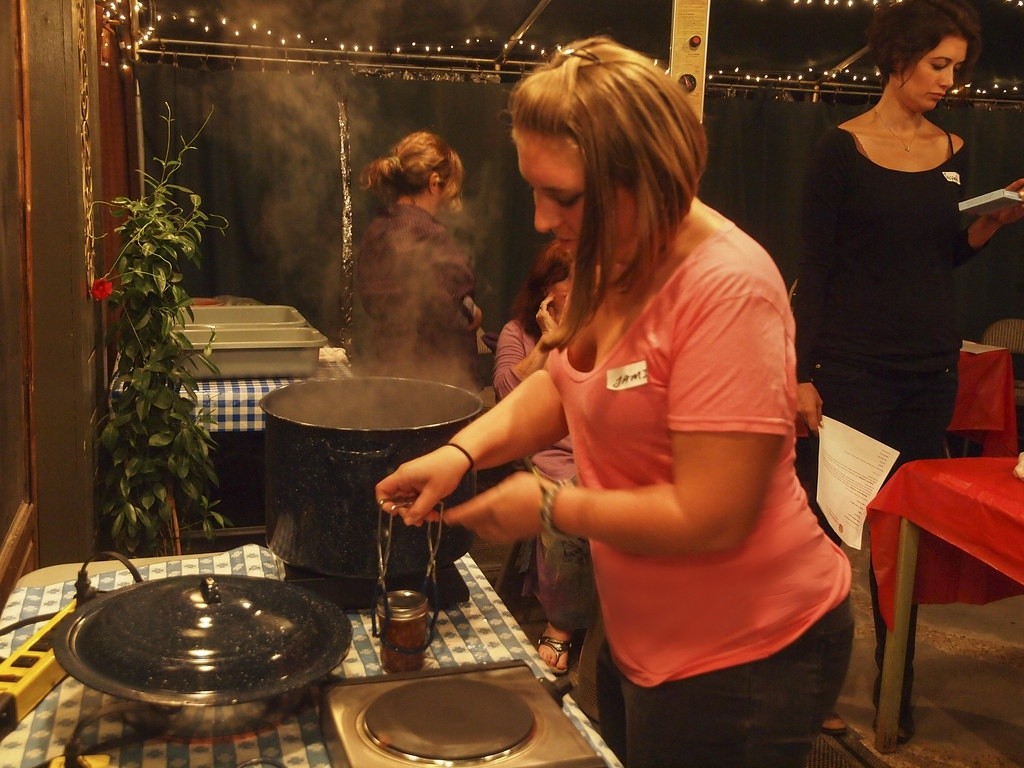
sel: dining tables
[862,457,1024,756]
[110,339,360,434]
[0,543,621,768]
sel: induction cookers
[308,646,606,767]
[281,549,470,617]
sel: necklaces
[873,105,921,152]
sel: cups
[377,590,429,674]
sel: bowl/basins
[109,680,296,735]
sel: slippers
[537,636,573,674]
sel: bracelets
[526,465,578,549]
[444,442,476,470]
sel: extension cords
[0,589,111,728]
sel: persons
[349,131,483,392]
[386,35,860,768]
[491,238,576,404]
[787,1,1023,459]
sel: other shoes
[819,709,846,735]
[873,708,916,744]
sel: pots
[256,370,483,582]
